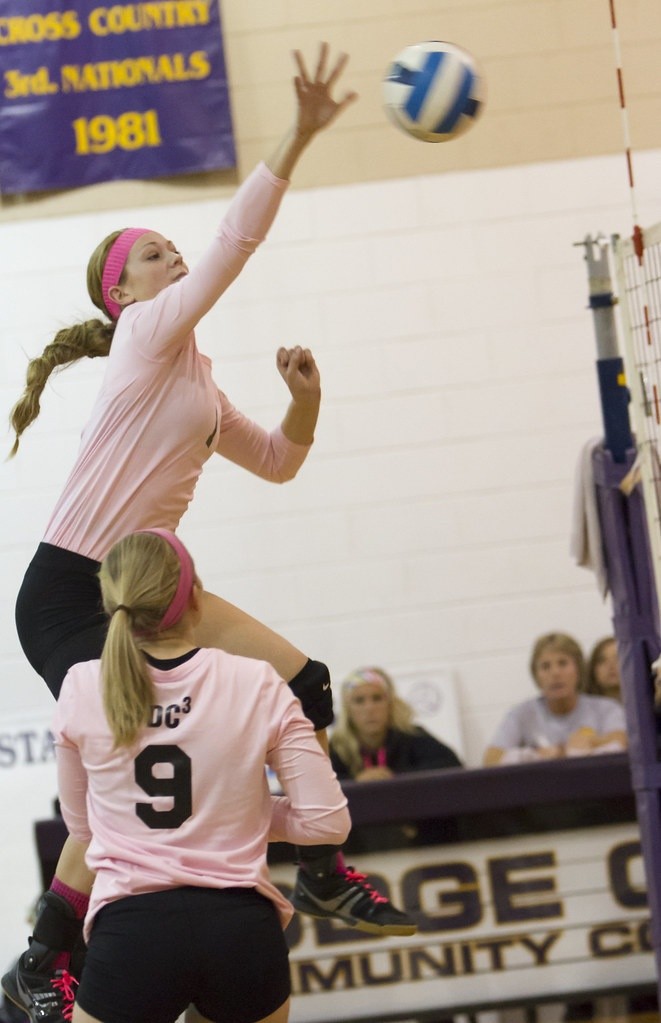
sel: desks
[36,752,661,1023]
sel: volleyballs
[381,40,486,143]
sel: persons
[57,529,353,1023]
[0,43,414,1023]
[484,633,628,768]
[329,666,463,784]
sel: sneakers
[0,949,81,1023]
[287,864,417,936]
